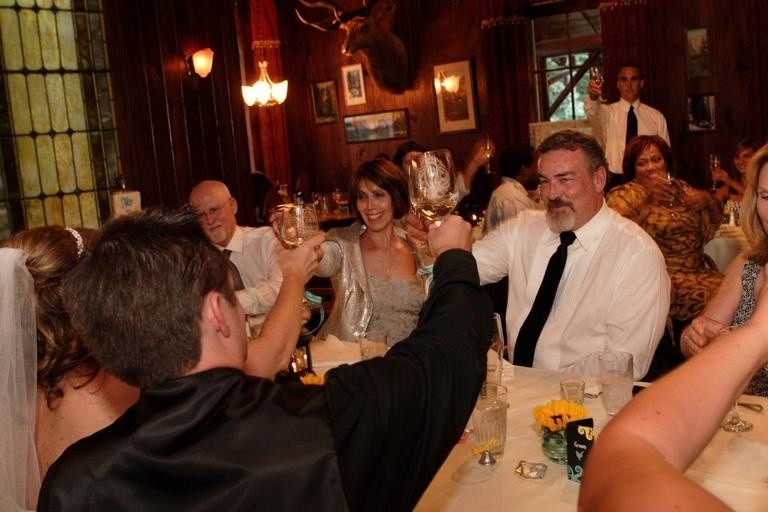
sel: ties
[624,104,638,145]
[500,227,581,367]
[219,247,246,294]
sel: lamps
[186,49,213,78]
[239,0,289,108]
[436,71,460,91]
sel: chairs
[704,253,719,271]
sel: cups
[248,311,268,341]
[472,311,634,458]
[359,332,387,360]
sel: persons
[0,224,328,510]
[283,158,431,347]
[576,265,767,512]
[39,214,491,511]
[394,64,768,393]
[180,181,288,334]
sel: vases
[543,432,567,463]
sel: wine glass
[407,148,459,227]
[268,191,329,247]
[717,326,755,432]
[661,169,679,208]
[709,153,721,191]
[479,134,494,176]
[587,65,609,104]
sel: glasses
[195,197,231,219]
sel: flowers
[299,373,323,385]
[534,399,587,432]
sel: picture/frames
[342,108,409,143]
[684,24,711,79]
[432,56,479,136]
[685,92,719,135]
[310,80,338,124]
[341,63,365,106]
